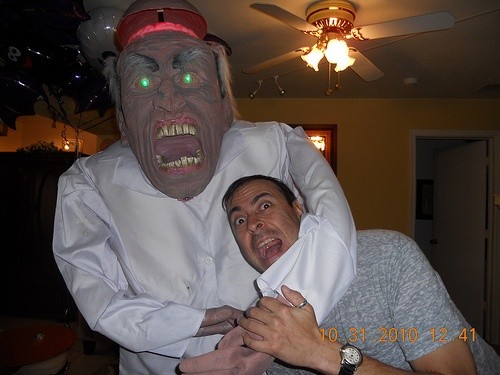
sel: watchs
[338,342,363,375]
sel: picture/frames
[289,123,337,181]
[416,177,433,222]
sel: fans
[241,0,460,98]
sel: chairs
[0,158,78,375]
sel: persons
[222,175,500,375]
[55,6,360,375]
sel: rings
[296,300,308,307]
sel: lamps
[300,33,357,96]
